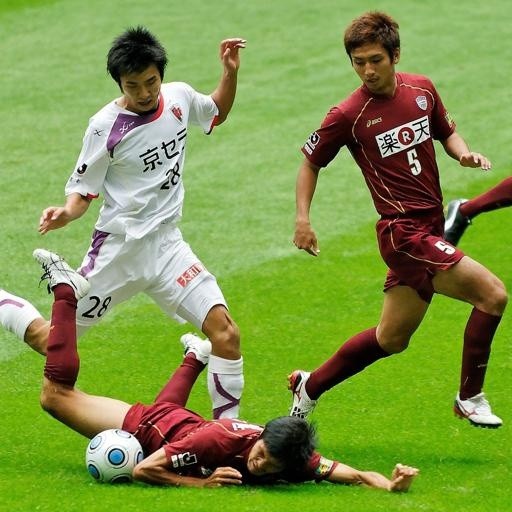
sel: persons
[34,246,422,494]
[0,22,246,422]
[287,10,511,429]
[441,173,512,248]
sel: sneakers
[443,199,470,246]
[32,250,89,301]
[287,369,317,425]
[454,391,502,429]
[180,334,213,365]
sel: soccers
[85,429,144,483]
